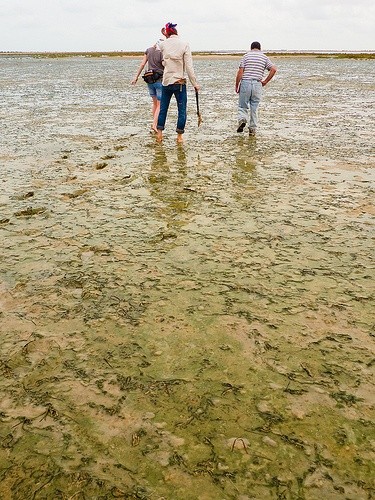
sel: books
[237,80,241,92]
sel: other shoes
[236,119,246,133]
[248,127,256,136]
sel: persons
[235,42,276,136]
[132,39,164,133]
[155,22,200,142]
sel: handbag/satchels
[142,69,163,84]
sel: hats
[161,22,178,38]
